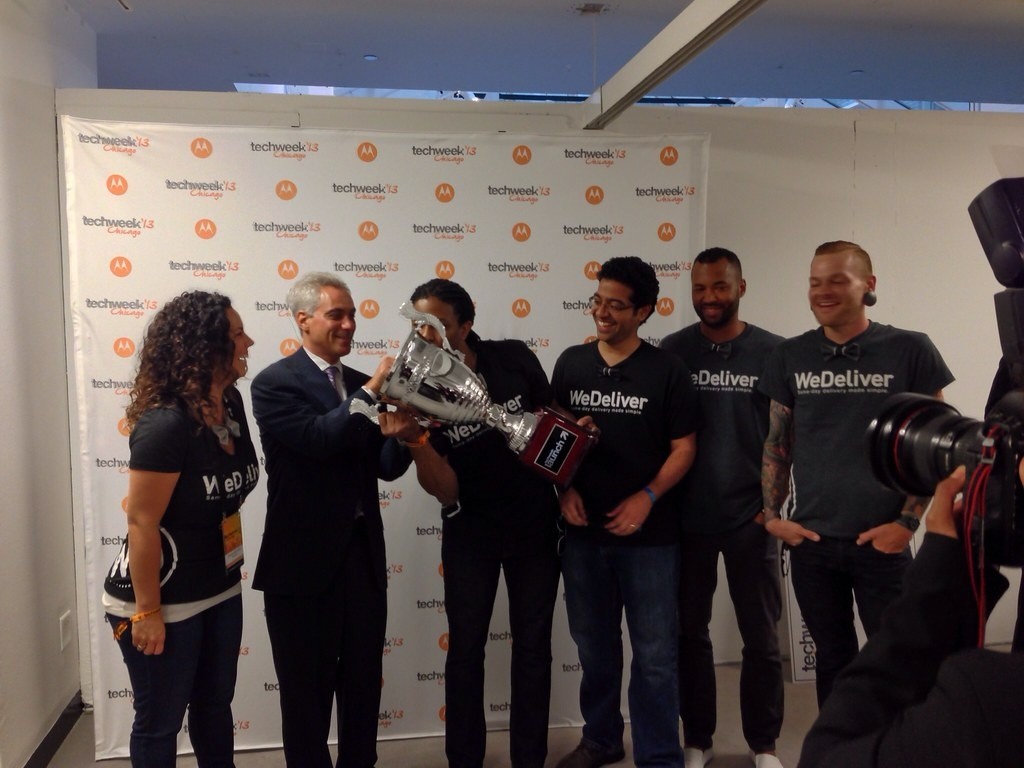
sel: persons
[797,446,1024,768]
[379,279,598,768]
[550,256,702,768]
[250,272,415,768]
[658,247,795,768]
[102,291,260,768]
[761,240,953,768]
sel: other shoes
[554,741,625,768]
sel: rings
[630,524,636,527]
[138,645,143,649]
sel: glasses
[588,297,636,313]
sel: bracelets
[130,608,162,623]
[896,512,919,532]
[405,431,427,448]
[645,487,656,506]
[763,513,781,521]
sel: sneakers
[682,746,713,768]
[747,749,784,768]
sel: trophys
[349,301,596,492]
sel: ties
[325,367,337,392]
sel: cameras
[861,178,1024,530]
